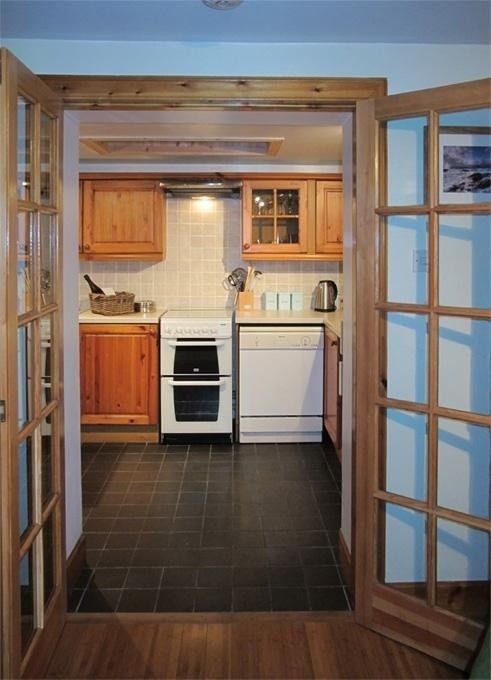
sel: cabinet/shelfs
[78,323,159,425]
[323,326,342,464]
[239,179,343,261]
[78,179,166,261]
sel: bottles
[84,274,105,295]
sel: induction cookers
[160,310,233,338]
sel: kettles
[315,279,338,312]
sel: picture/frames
[423,124,491,217]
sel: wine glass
[278,191,295,215]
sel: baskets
[89,288,136,316]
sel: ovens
[159,339,233,446]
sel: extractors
[159,180,242,201]
